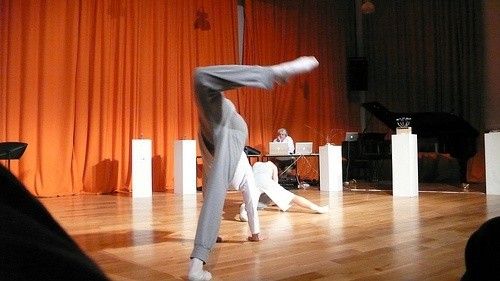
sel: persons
[272,128,294,175]
[187,56,319,281]
[239,160,330,222]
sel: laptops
[345,132,358,141]
[295,142,313,154]
[268,142,288,155]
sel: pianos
[342,102,480,187]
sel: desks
[244,154,320,188]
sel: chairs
[0,142,28,170]
[267,157,298,185]
